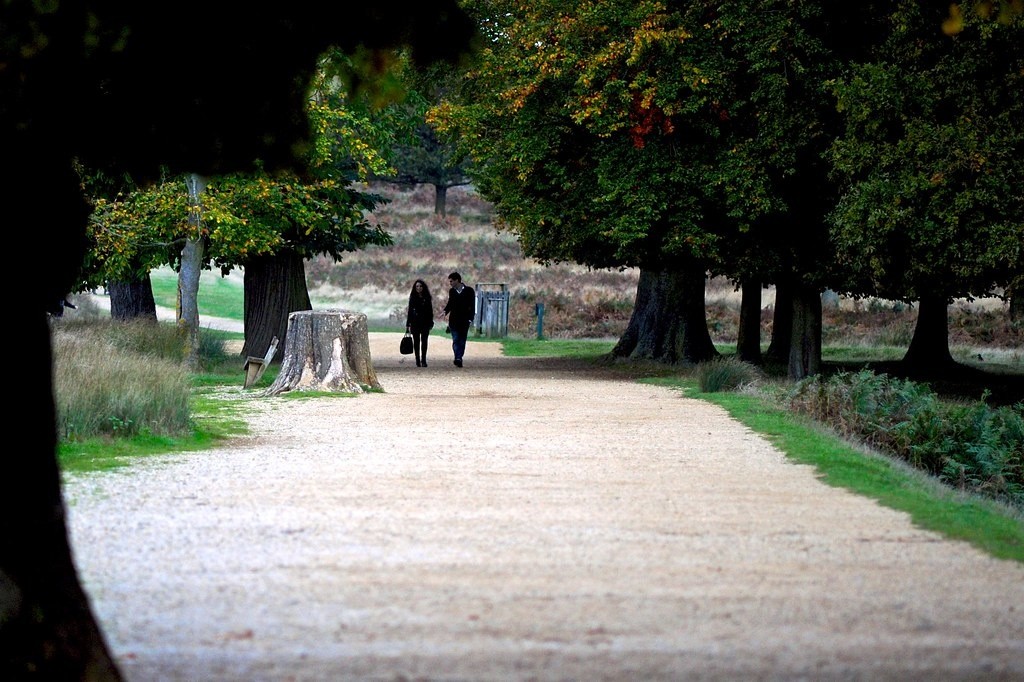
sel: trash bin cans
[475,283,510,339]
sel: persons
[437,271,475,367]
[405,278,433,367]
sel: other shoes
[416,360,420,367]
[454,358,462,367]
[422,360,427,367]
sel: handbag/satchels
[400,331,413,354]
